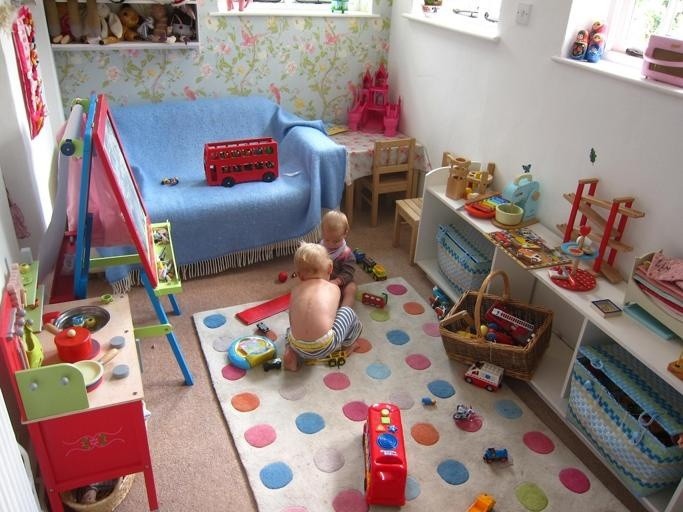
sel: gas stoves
[54,336,129,393]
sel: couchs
[104,95,346,296]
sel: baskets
[438,270,554,383]
[61,473,135,512]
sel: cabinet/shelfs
[416,163,683,511]
[45,1,199,50]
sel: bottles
[18,263,32,285]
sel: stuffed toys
[44,1,196,44]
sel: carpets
[191,277,629,511]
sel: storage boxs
[569,340,682,500]
[434,222,492,296]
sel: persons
[284,242,364,372]
[319,210,357,308]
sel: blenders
[490,173,541,229]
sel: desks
[1,259,158,512]
[323,122,431,229]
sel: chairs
[358,139,413,225]
[392,151,472,267]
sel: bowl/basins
[53,304,110,335]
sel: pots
[45,323,92,360]
[72,348,116,387]
